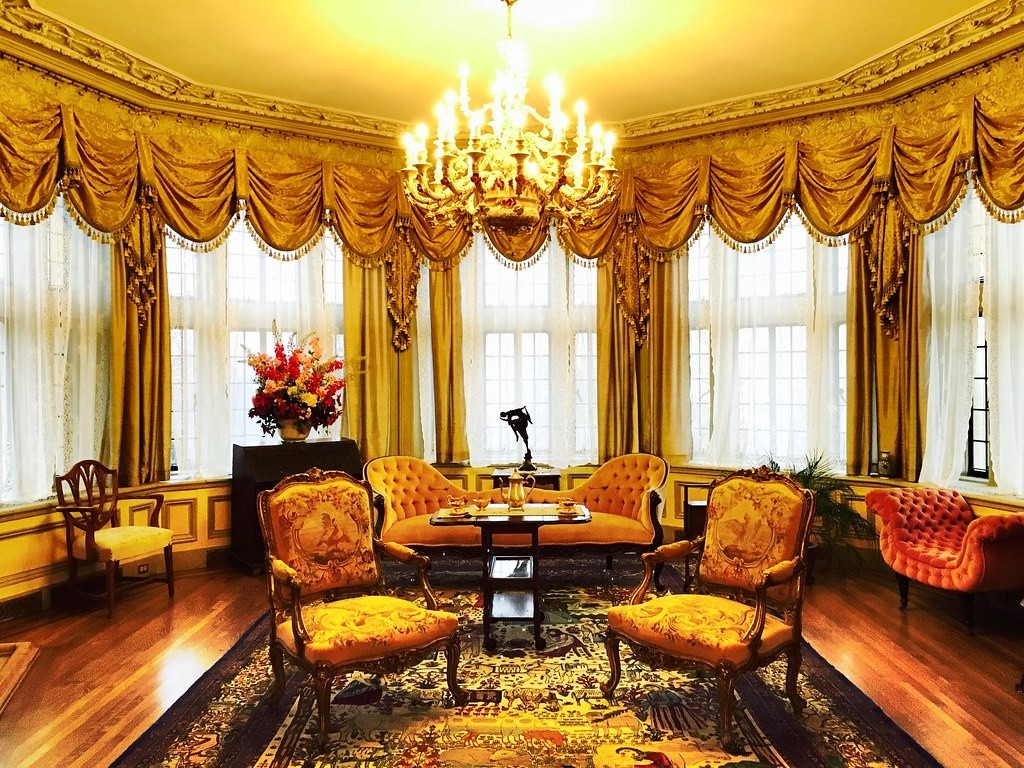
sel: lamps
[400,0,617,232]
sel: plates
[556,507,579,514]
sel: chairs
[256,467,468,754]
[55,459,175,621]
[602,465,816,753]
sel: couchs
[360,451,669,611]
[865,483,1024,637]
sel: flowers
[236,320,353,437]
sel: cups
[557,495,578,513]
[446,494,467,514]
[472,498,492,512]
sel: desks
[430,504,591,652]
[491,467,561,492]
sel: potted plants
[761,446,877,580]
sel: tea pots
[499,467,536,513]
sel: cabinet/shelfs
[232,437,364,576]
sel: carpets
[105,555,947,768]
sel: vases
[278,417,311,443]
[878,450,892,480]
